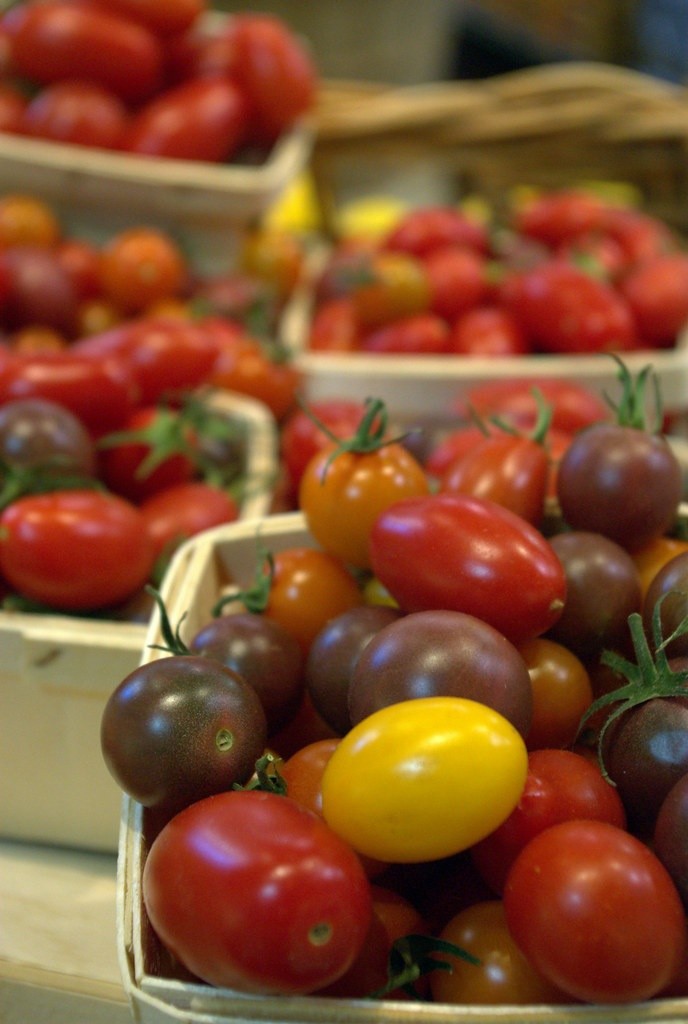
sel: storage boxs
[0,391,277,853]
[117,510,688,1024]
[279,244,688,501]
[0,12,315,278]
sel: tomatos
[0,0,687,1005]
